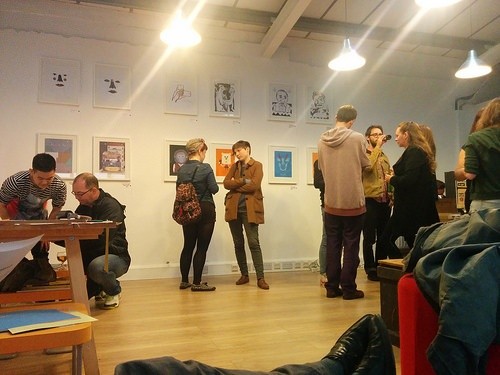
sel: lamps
[455,0,493,78]
[328,0,368,71]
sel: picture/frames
[266,81,299,120]
[91,136,132,181]
[38,56,83,106]
[209,74,241,119]
[36,133,78,180]
[307,146,321,185]
[304,85,335,123]
[164,139,192,183]
[93,62,131,110]
[267,144,298,185]
[162,74,200,116]
[207,141,242,183]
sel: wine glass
[57,252,67,270]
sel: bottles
[382,135,391,140]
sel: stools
[0,267,95,375]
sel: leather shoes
[321,313,396,375]
[236,275,249,285]
[257,278,270,289]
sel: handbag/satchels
[224,192,230,205]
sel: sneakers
[179,282,192,289]
[95,291,122,300]
[103,294,119,309]
[191,282,216,291]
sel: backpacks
[172,163,201,226]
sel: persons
[455,95,500,213]
[362,126,396,281]
[112,313,397,375]
[383,121,441,259]
[317,105,365,300]
[55,173,131,308]
[0,153,67,280]
[223,140,270,289]
[314,160,328,285]
[176,138,219,292]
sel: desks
[376,258,410,348]
[0,214,119,375]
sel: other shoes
[326,288,343,298]
[320,273,328,288]
[367,272,380,281]
[343,289,365,300]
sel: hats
[437,180,447,189]
[185,139,205,154]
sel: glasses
[370,133,383,137]
[232,148,243,156]
[71,186,95,198]
